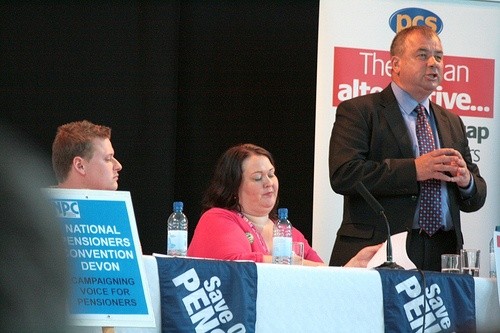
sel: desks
[69,255,500,333]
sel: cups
[292,241,305,265]
[441,253,461,274]
[460,249,481,277]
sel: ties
[414,104,441,237]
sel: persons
[52,119,123,191]
[186,143,326,266]
[0,158,71,333]
[328,25,487,273]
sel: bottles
[272,208,292,265]
[490,238,496,278]
[166,201,188,256]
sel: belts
[413,227,455,238]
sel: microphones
[355,183,405,269]
